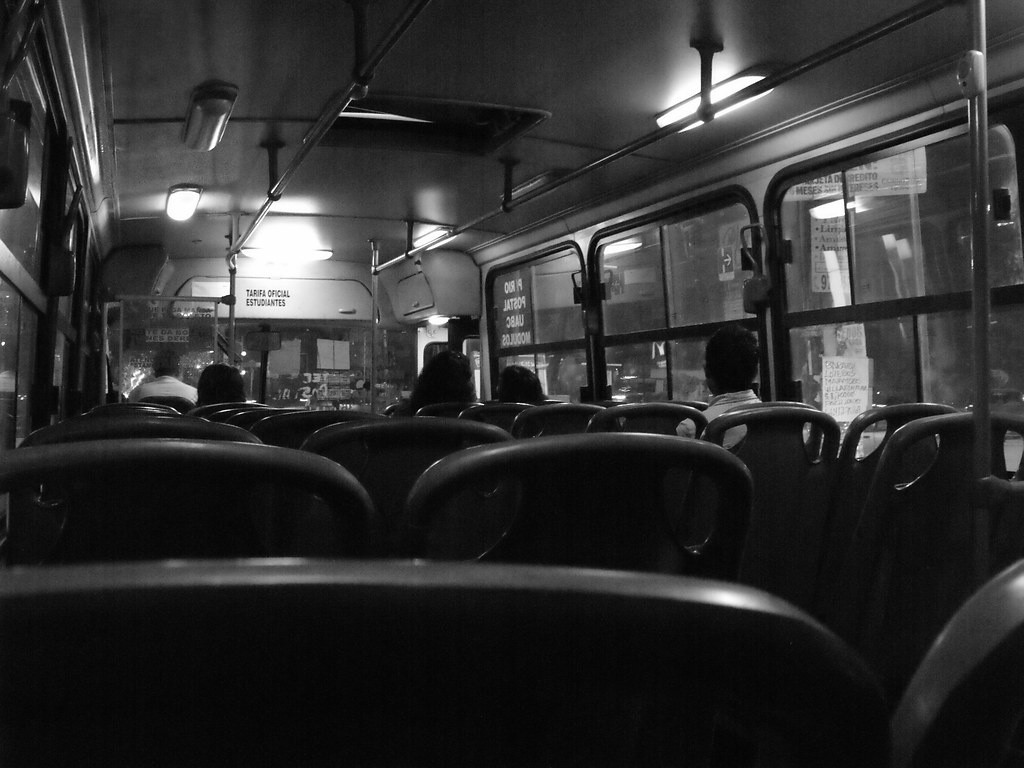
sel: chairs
[0,398,1024,768]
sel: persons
[392,351,477,417]
[676,327,762,449]
[196,364,246,406]
[128,350,198,403]
[498,366,548,407]
